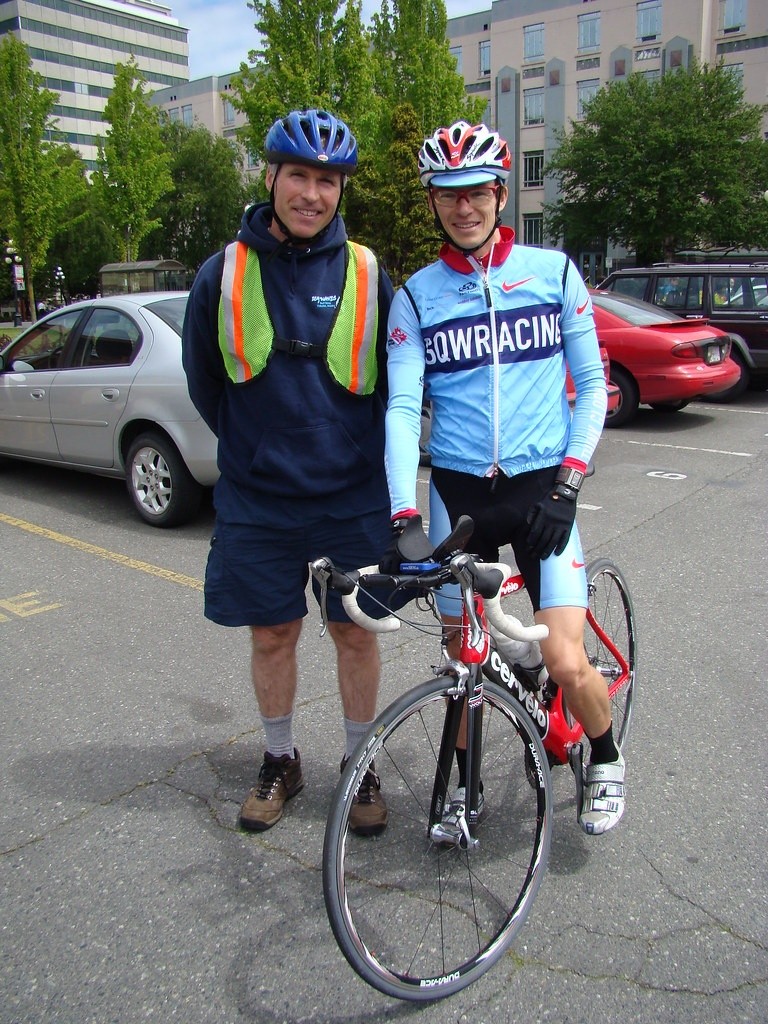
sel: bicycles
[310,550,637,1002]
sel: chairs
[96,329,135,364]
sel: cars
[408,289,742,466]
[0,291,222,528]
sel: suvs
[593,250,768,404]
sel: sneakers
[340,753,388,836]
[238,747,303,831]
[430,787,484,843]
[579,740,625,835]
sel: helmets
[264,109,358,174]
[417,120,512,188]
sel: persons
[655,273,738,311]
[182,112,395,833]
[37,300,46,319]
[384,123,626,843]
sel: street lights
[5,239,26,328]
[53,266,65,303]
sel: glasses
[430,185,500,208]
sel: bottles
[491,615,549,684]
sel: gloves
[379,527,416,575]
[526,481,578,560]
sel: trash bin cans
[14,314,22,327]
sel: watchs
[555,467,585,491]
